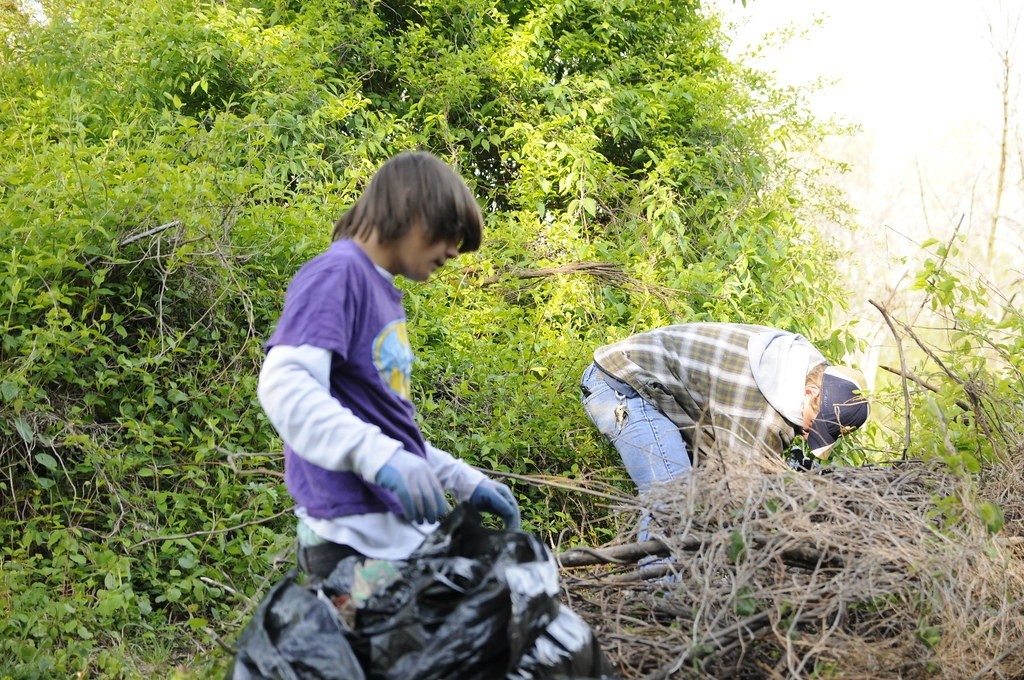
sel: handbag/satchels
[224,498,613,680]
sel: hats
[807,373,869,460]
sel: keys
[614,390,627,430]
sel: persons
[580,323,868,595]
[257,152,520,573]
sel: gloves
[377,448,446,524]
[469,478,521,529]
